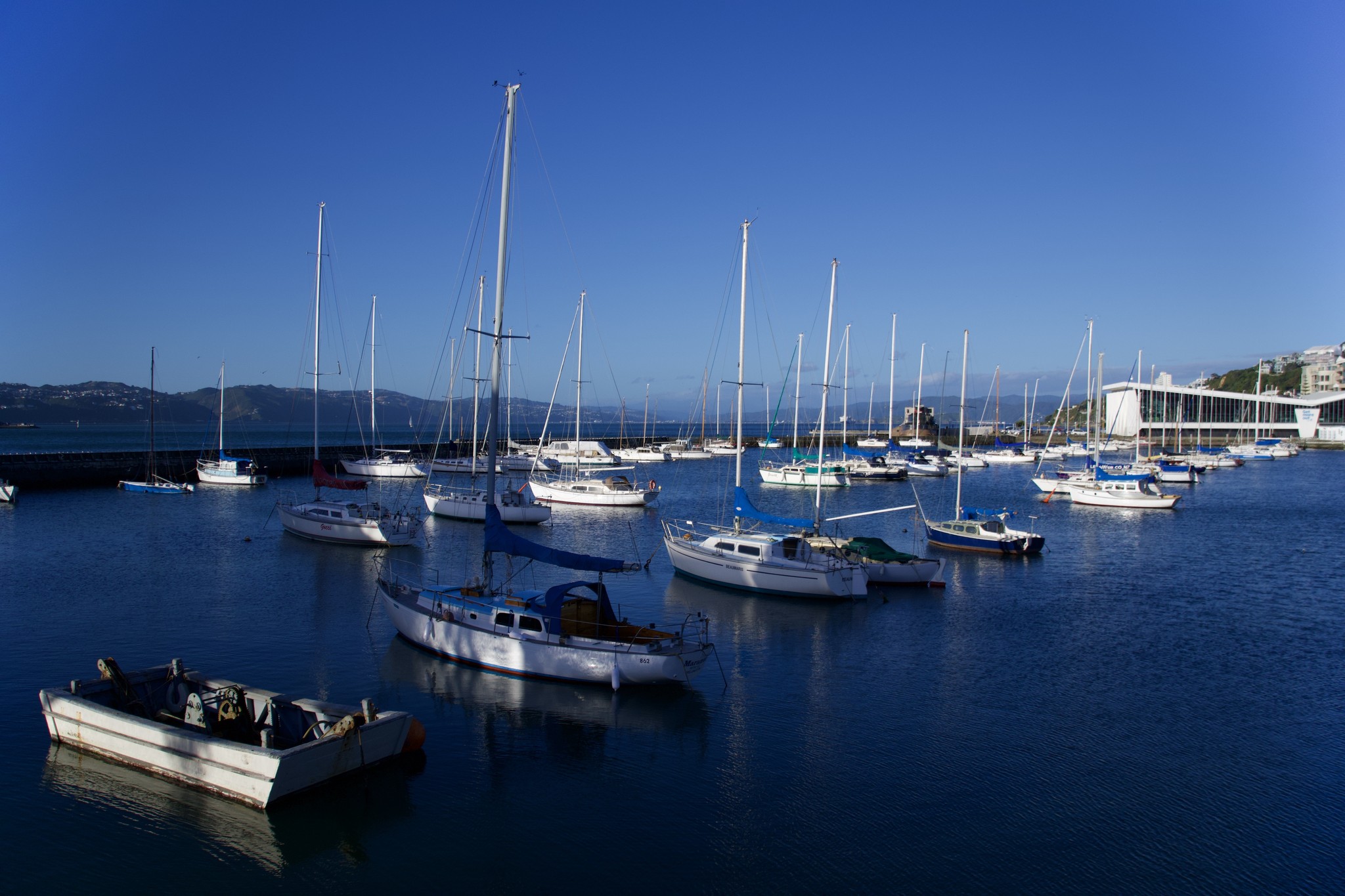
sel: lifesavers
[649,480,656,490]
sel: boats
[40,654,426,812]
[0,478,19,502]
[38,742,322,877]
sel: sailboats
[373,81,723,689]
[437,274,1304,509]
[783,256,951,586]
[118,347,200,495]
[525,291,663,508]
[340,295,439,477]
[660,212,876,603]
[422,274,556,525]
[197,362,270,486]
[277,200,435,550]
[906,329,1054,557]
